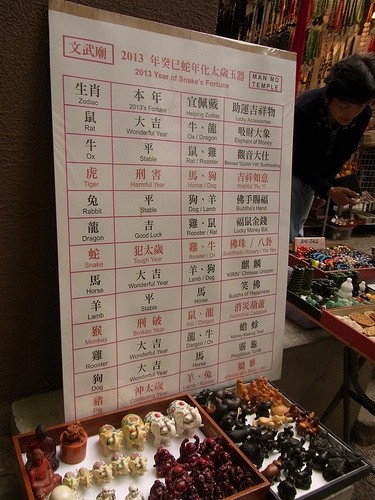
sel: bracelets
[297,245,375,270]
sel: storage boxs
[192,379,372,500]
[287,242,375,363]
[325,222,357,241]
[12,391,270,500]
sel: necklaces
[217,0,375,93]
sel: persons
[24,423,60,475]
[290,53,375,244]
[146,434,256,500]
[95,486,117,500]
[27,448,62,500]
[125,484,146,500]
[43,485,83,500]
